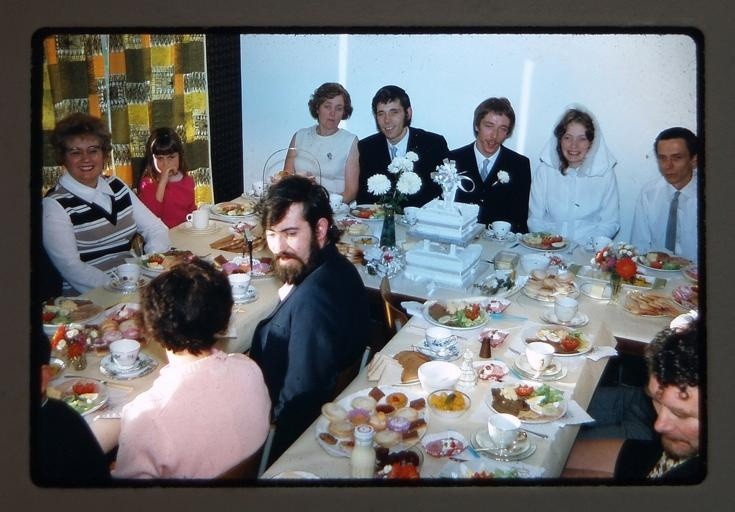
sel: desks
[42,195,697,478]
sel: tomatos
[650,262,662,270]
[562,338,580,350]
[149,255,163,264]
[515,385,533,396]
[464,303,480,319]
[66,343,83,358]
[43,311,55,321]
[72,382,96,393]
[358,212,373,218]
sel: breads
[627,292,690,317]
[210,234,268,253]
[646,253,671,264]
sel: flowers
[490,168,514,189]
[369,147,421,214]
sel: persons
[567,316,700,483]
[574,309,700,445]
[111,256,271,479]
[139,128,197,228]
[43,113,170,297]
[527,109,621,247]
[249,176,371,465]
[38,332,111,480]
[448,98,531,234]
[630,127,697,265]
[357,85,448,215]
[283,84,360,205]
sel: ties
[390,147,397,159]
[665,191,680,254]
[480,159,489,182]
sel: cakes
[93,305,148,356]
[213,255,275,275]
[523,268,577,301]
[319,387,427,453]
[406,198,482,283]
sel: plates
[478,252,699,303]
[350,204,389,221]
[518,232,570,251]
[52,378,109,417]
[316,380,567,470]
[231,286,257,305]
[618,296,671,319]
[40,297,104,330]
[269,469,319,478]
[381,297,595,386]
[484,231,517,243]
[110,276,150,292]
[212,201,256,218]
[99,353,158,377]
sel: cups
[108,338,139,369]
[228,273,250,295]
[488,220,511,240]
[185,210,210,230]
[243,181,267,199]
[116,263,139,286]
[403,207,418,226]
[330,194,342,210]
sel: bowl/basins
[345,223,379,247]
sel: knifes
[210,218,234,225]
[63,375,132,393]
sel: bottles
[350,424,376,479]
